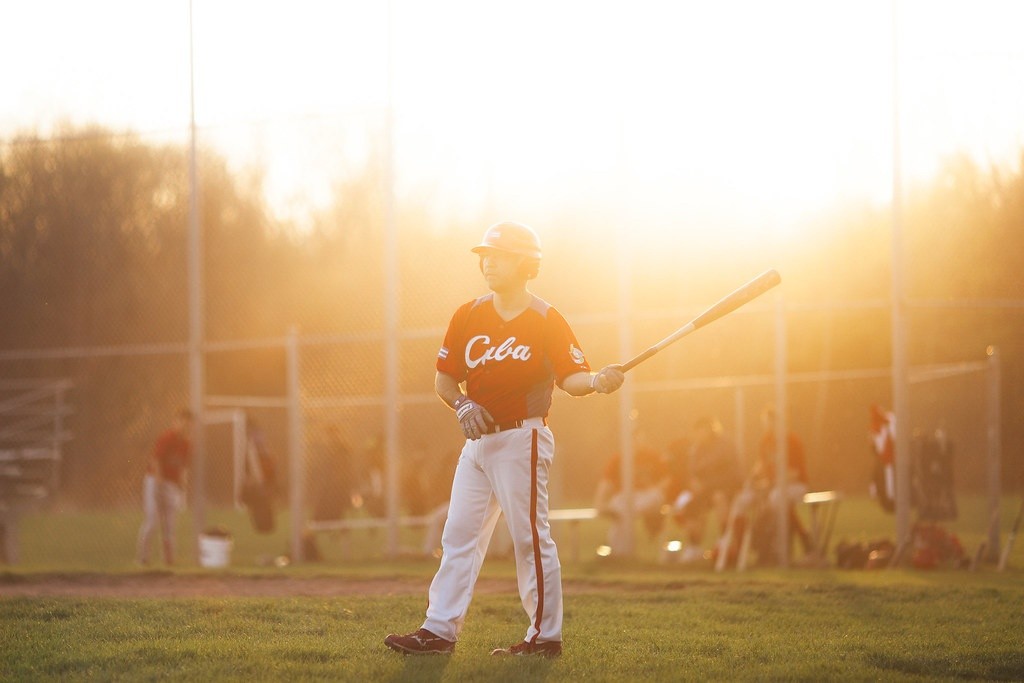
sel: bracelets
[590,372,597,391]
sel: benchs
[302,490,840,566]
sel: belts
[478,415,547,435]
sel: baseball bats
[591,265,784,398]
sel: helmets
[471,221,542,280]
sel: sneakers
[384,628,456,656]
[491,641,563,659]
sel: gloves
[454,395,494,441]
[591,364,624,395]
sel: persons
[384,221,624,655]
[137,407,814,570]
